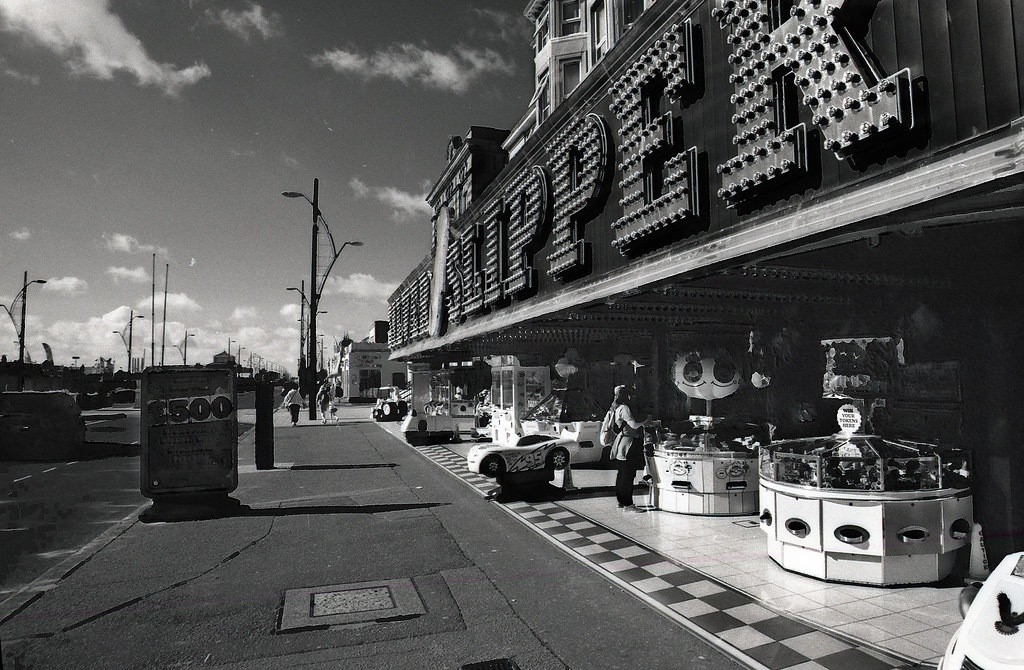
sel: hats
[614,386,637,398]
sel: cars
[467,433,581,477]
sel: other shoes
[289,421,296,428]
[321,418,327,425]
[617,505,648,514]
[330,416,340,425]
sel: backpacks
[599,410,615,447]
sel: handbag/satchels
[285,404,289,410]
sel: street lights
[113,310,145,372]
[238,344,246,365]
[285,279,328,411]
[173,330,195,365]
[282,189,366,419]
[226,337,236,362]
[0,271,47,392]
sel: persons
[610,384,650,513]
[281,380,294,397]
[284,383,307,427]
[328,397,339,423]
[299,385,306,409]
[316,385,329,425]
